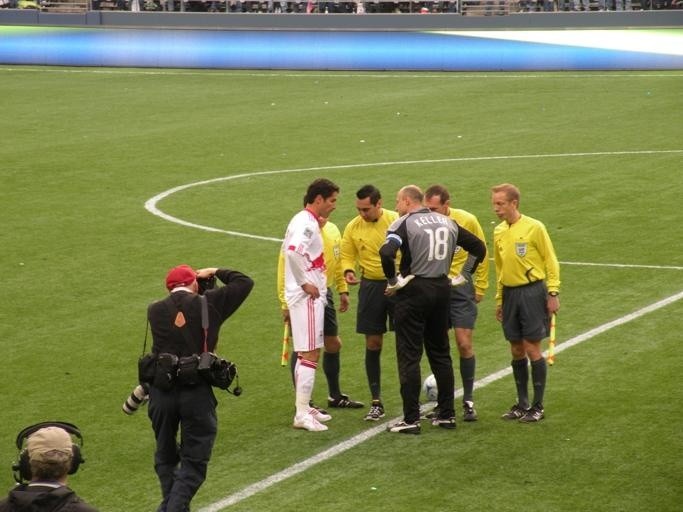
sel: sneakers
[306,407,332,423]
[292,412,329,433]
[385,419,421,434]
[500,403,531,421]
[462,400,480,423]
[430,415,457,429]
[420,406,442,422]
[363,399,385,422]
[307,400,327,415]
[517,403,544,423]
[326,393,365,408]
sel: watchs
[548,291,559,296]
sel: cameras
[197,276,216,294]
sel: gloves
[384,272,415,292]
[447,273,467,288]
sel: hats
[163,263,197,290]
[24,426,73,464]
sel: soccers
[423,374,438,401]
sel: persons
[0,426,99,512]
[423,184,490,422]
[147,263,256,512]
[377,183,486,435]
[277,193,366,415]
[281,177,340,433]
[0,0,683,15]
[339,183,402,422]
[490,183,561,424]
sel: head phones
[12,422,84,480]
